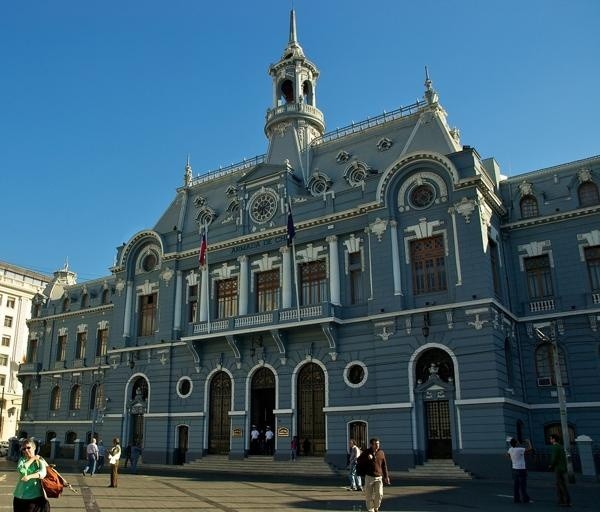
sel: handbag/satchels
[43,464,63,498]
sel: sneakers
[82,470,118,488]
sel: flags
[199,230,207,271]
[286,203,296,249]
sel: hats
[112,438,120,443]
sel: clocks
[245,187,279,227]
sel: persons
[344,438,363,492]
[545,434,574,506]
[105,438,122,487]
[506,438,536,504]
[130,441,144,473]
[250,424,260,455]
[81,437,99,478]
[123,442,133,468]
[264,425,274,456]
[291,435,298,462]
[96,438,107,473]
[11,438,51,512]
[356,437,390,511]
